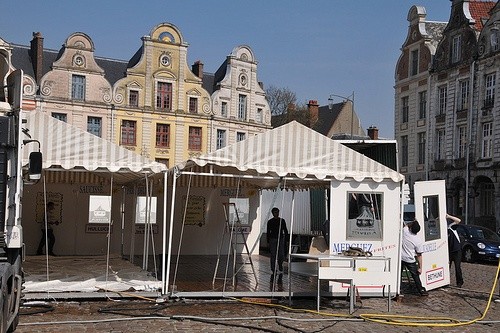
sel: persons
[36,202,60,256]
[436,213,465,289]
[400,220,430,297]
[265,207,290,274]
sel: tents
[165,119,406,298]
[15,108,165,298]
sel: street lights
[328,92,355,140]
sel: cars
[451,224,500,264]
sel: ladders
[223,202,258,286]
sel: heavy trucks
[0,45,43,333]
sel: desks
[290,254,392,314]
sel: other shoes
[48,252,57,256]
[458,281,463,288]
[279,264,283,271]
[420,291,429,296]
[272,266,275,271]
